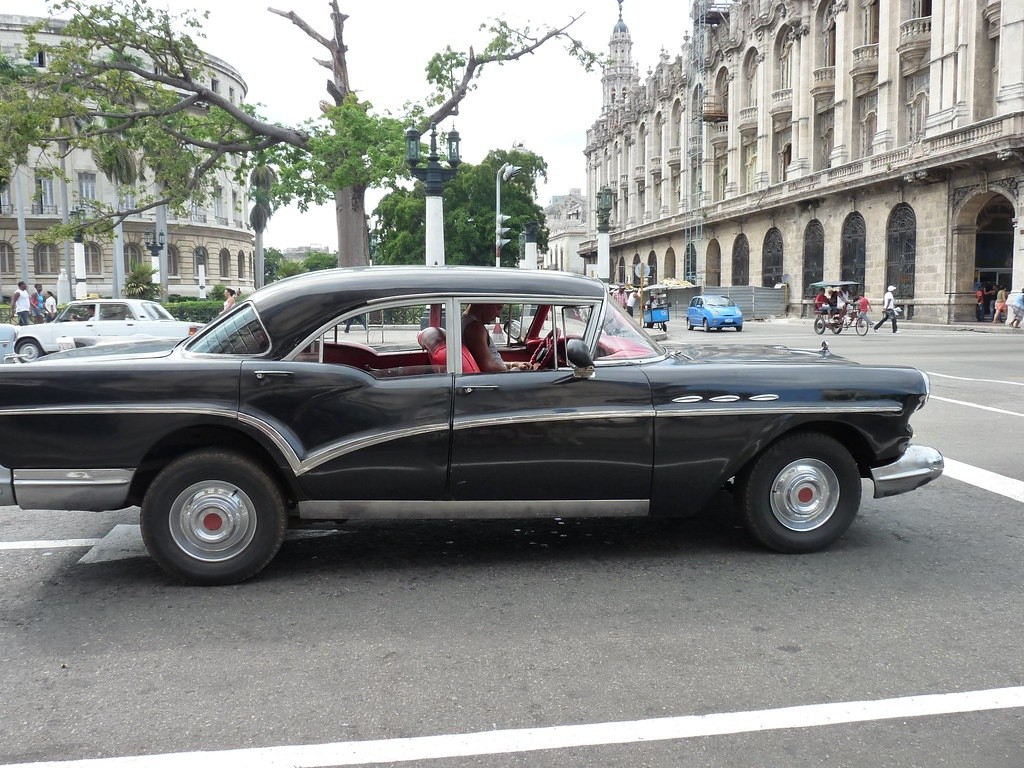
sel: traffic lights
[497,214,512,249]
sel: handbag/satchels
[887,310,894,319]
[30,292,39,307]
[822,303,829,308]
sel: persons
[873,285,903,333]
[30,283,52,324]
[219,288,235,314]
[612,284,657,317]
[815,285,875,329]
[461,304,533,373]
[237,288,241,296]
[342,315,371,334]
[45,291,57,323]
[10,282,34,326]
[1009,288,1024,328]
[976,284,1008,324]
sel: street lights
[69,204,87,300]
[595,185,612,284]
[144,227,165,300]
[405,117,462,331]
[492,162,523,345]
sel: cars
[0,298,206,366]
[0,264,945,586]
[686,294,744,332]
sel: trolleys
[641,285,669,331]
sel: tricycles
[808,280,869,336]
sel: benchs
[417,326,479,374]
[813,304,842,314]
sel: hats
[620,284,626,288]
[888,286,897,291]
[651,296,656,299]
